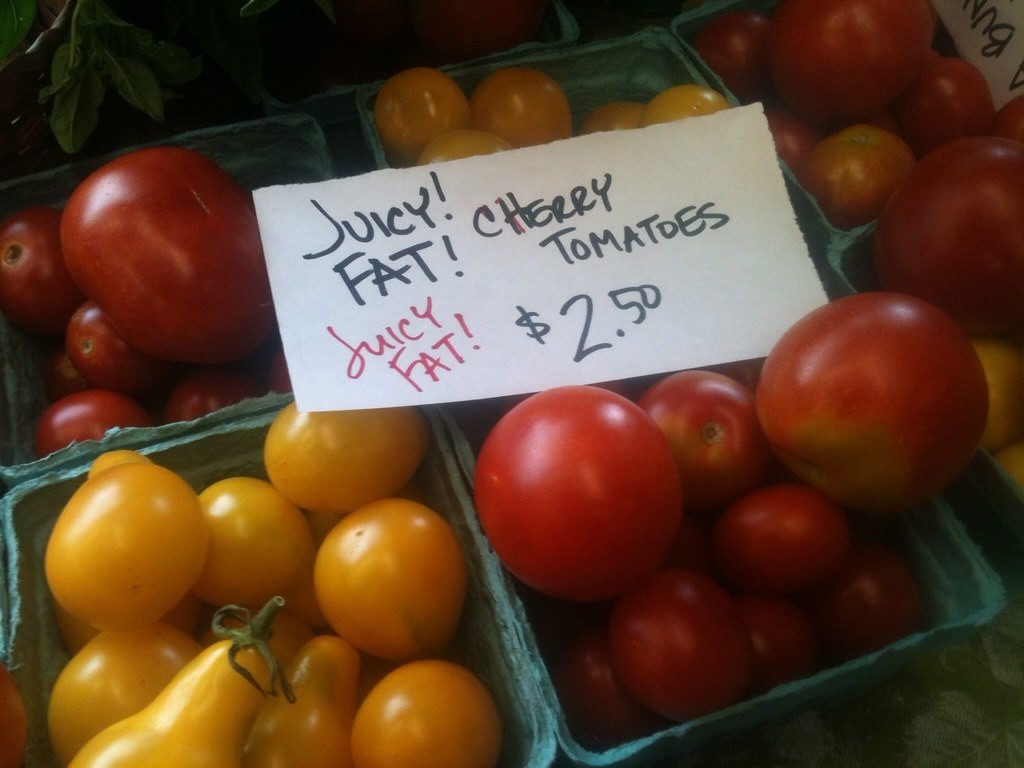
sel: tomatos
[0,0,1024,768]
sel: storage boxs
[0,1,1021,767]
